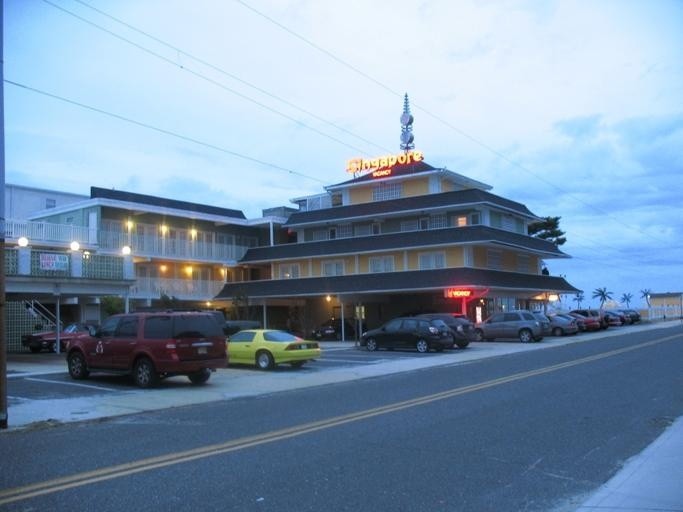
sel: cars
[225,320,262,335]
[311,317,364,340]
[473,308,640,343]
[21,323,100,354]
[226,327,320,370]
[358,312,478,354]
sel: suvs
[205,310,227,333]
[65,307,223,390]
